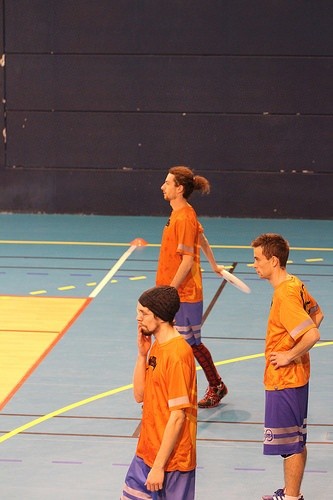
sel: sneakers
[198,382,228,408]
[262,487,304,500]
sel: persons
[118,285,197,500]
[250,233,324,500]
[155,165,229,408]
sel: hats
[138,285,180,322]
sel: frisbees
[218,269,251,295]
